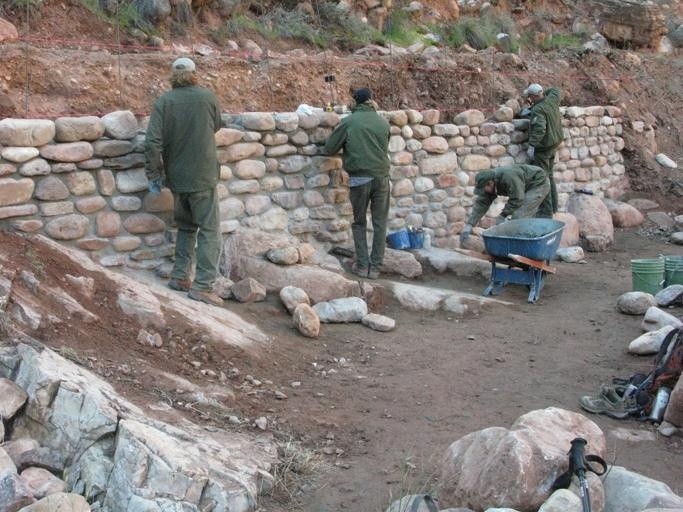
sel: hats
[353,87,372,105]
[472,170,496,196]
[523,83,543,97]
[170,56,198,76]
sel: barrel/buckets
[631,257,665,295]
[387,230,409,249]
[409,230,425,249]
[665,254,683,291]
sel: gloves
[460,224,472,242]
[525,145,535,162]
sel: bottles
[423,231,431,250]
[650,384,671,422]
[408,223,423,233]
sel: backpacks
[635,327,683,416]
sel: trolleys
[454,217,567,304]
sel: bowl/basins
[334,105,348,114]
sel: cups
[322,106,333,112]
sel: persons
[459,164,551,243]
[148,58,224,307]
[321,87,391,279]
[523,83,563,220]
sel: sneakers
[351,262,382,280]
[168,277,193,291]
[579,382,638,419]
[188,286,224,307]
